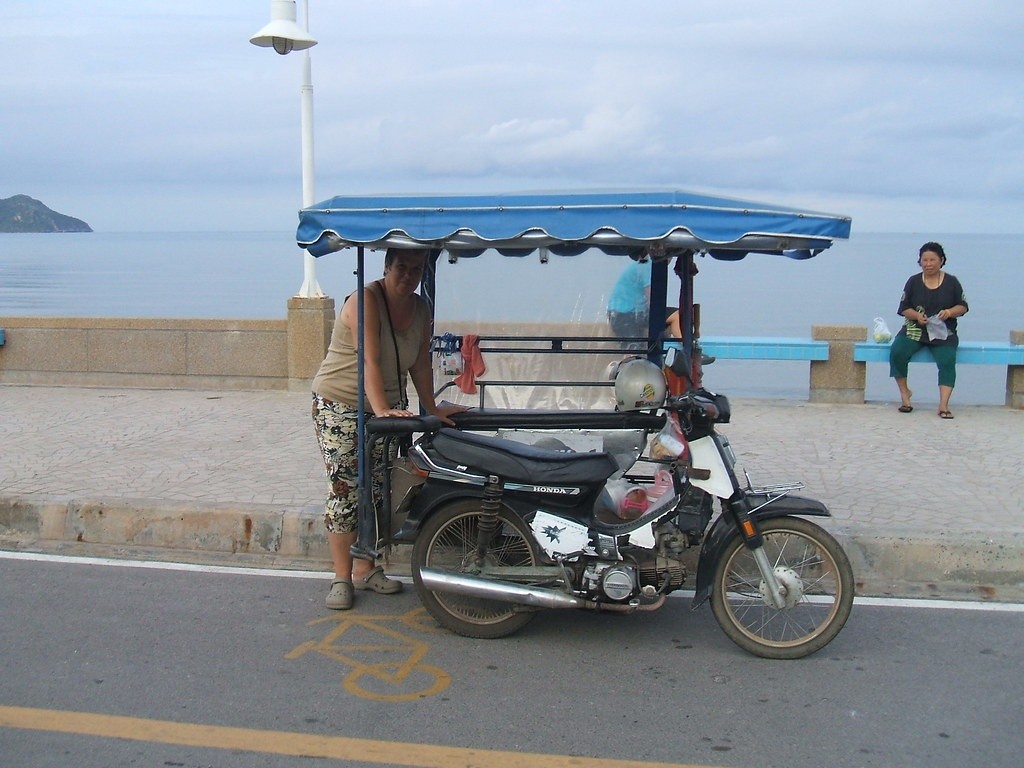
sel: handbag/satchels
[872,317,893,344]
[399,431,414,457]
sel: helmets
[608,355,668,412]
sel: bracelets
[946,308,952,317]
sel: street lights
[248,0,328,299]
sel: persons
[889,243,970,419]
[606,253,715,366]
[312,246,467,609]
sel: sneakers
[700,352,716,365]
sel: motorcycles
[293,184,856,661]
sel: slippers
[939,409,954,419]
[351,564,404,594]
[325,578,356,609]
[898,405,914,413]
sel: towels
[451,334,486,395]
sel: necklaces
[924,271,940,286]
